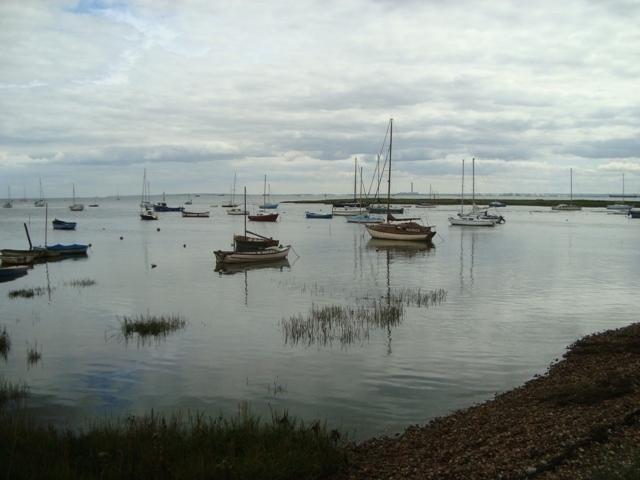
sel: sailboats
[332,118,502,241]
[213,263,293,306]
[137,163,291,263]
[363,239,438,353]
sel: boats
[552,167,582,210]
[0,174,89,278]
[89,204,99,207]
[608,167,634,210]
[305,211,333,218]
[629,208,640,218]
[489,201,505,207]
